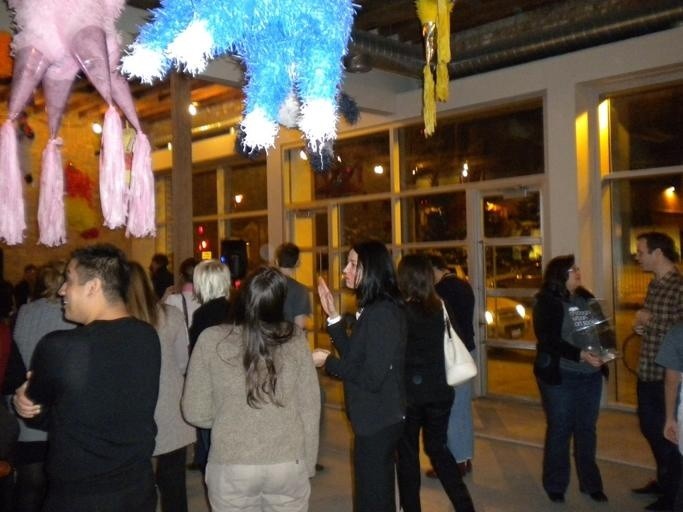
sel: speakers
[220,240,246,279]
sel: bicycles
[621,333,641,376]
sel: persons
[310,242,476,512]
[630,231,683,511]
[0,239,326,512]
[533,254,618,502]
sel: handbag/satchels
[443,326,478,387]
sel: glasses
[567,265,581,273]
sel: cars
[313,244,530,358]
[489,259,542,306]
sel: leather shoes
[591,491,609,503]
[426,457,474,478]
[630,480,664,499]
[548,491,566,503]
